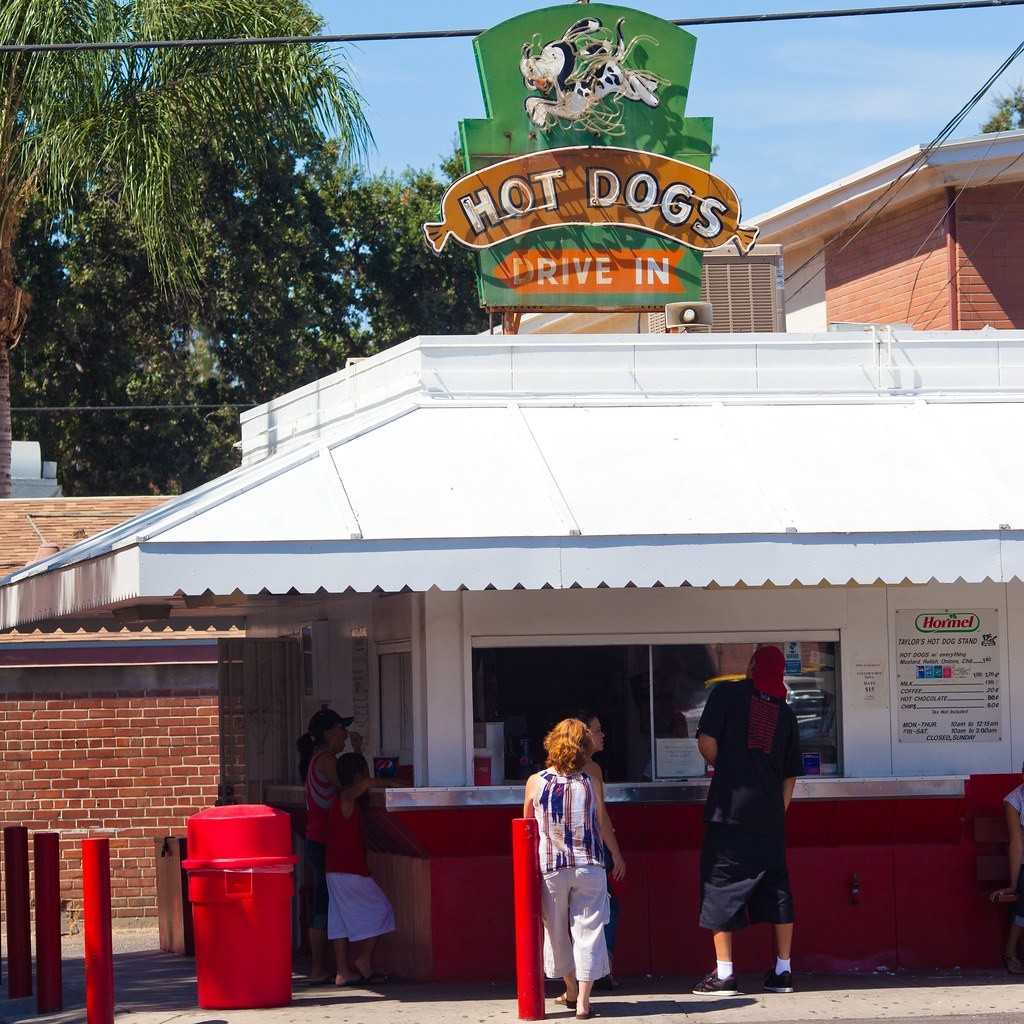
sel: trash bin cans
[177,801,299,1011]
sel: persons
[296,710,363,985]
[573,710,626,987]
[642,704,699,779]
[325,753,407,987]
[990,764,1024,974]
[523,718,626,1019]
[691,646,805,995]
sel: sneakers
[764,967,794,992]
[691,967,738,995]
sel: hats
[751,645,787,696]
[309,709,355,735]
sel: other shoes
[596,974,617,989]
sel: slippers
[575,1004,594,1019]
[554,994,577,1007]
[310,977,336,986]
[1003,953,1023,975]
[345,974,385,985]
